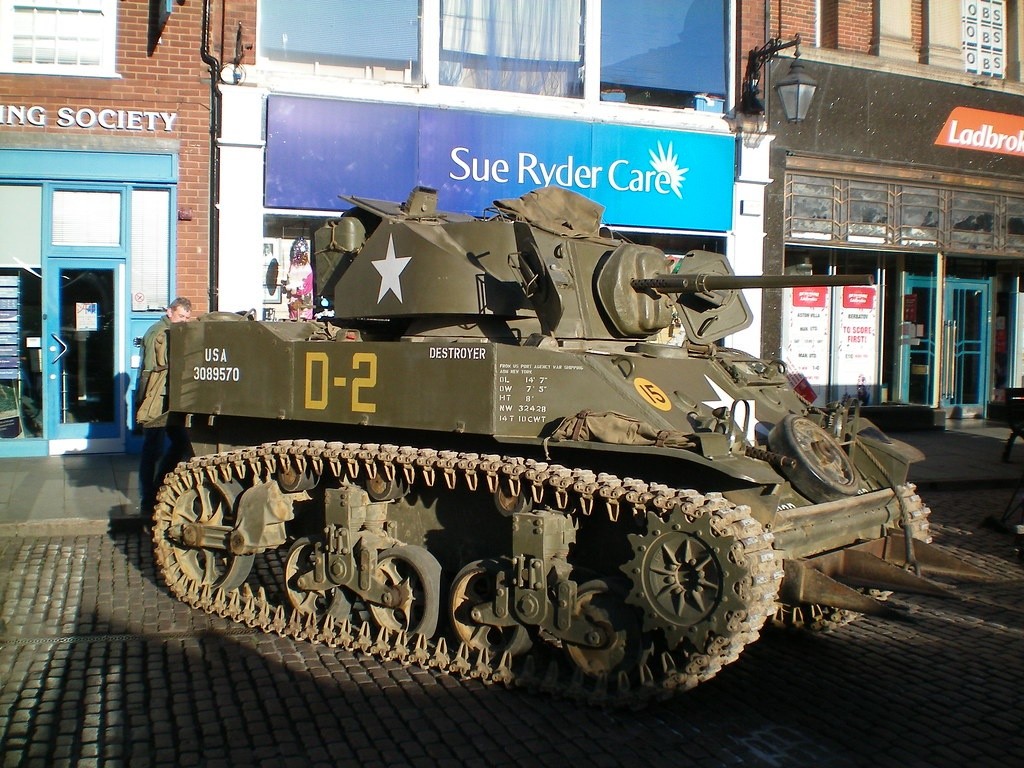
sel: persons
[135,295,192,515]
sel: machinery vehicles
[139,179,991,715]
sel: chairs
[1001,388,1024,462]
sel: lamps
[741,33,819,125]
